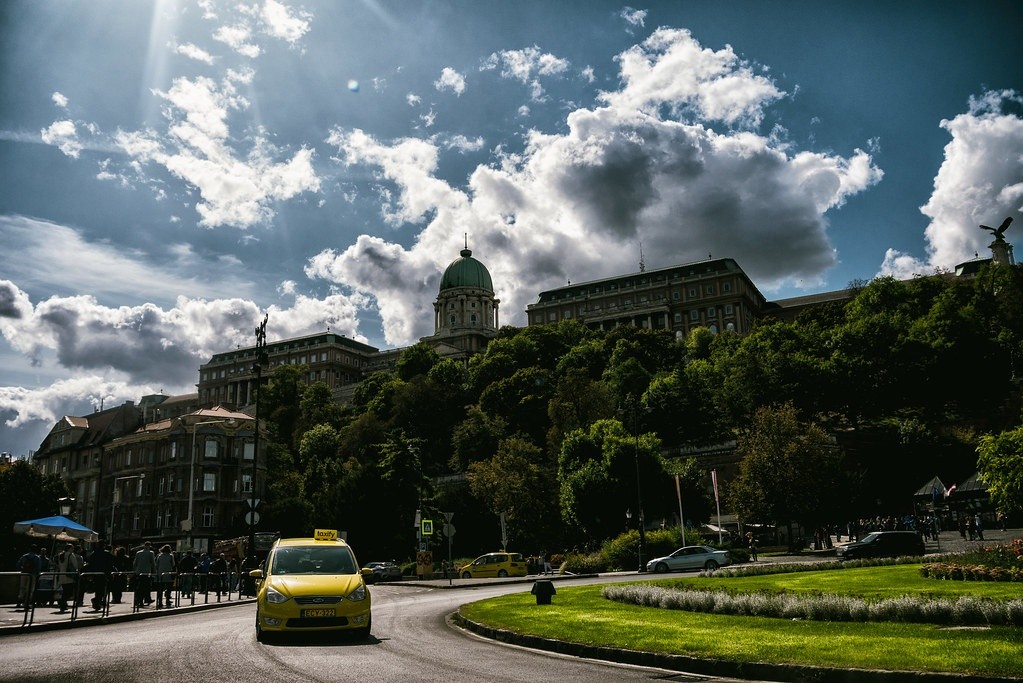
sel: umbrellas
[14,517,99,554]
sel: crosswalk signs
[422,520,433,534]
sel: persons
[538,548,552,573]
[733,532,755,548]
[999,509,1007,531]
[834,511,984,543]
[18,540,265,611]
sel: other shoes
[144,599,154,603]
[17,603,31,609]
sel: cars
[248,529,372,642]
[646,545,733,573]
[362,562,402,581]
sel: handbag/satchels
[65,564,78,579]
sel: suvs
[836,531,926,560]
[459,552,528,579]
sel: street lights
[615,391,656,573]
[238,314,271,592]
